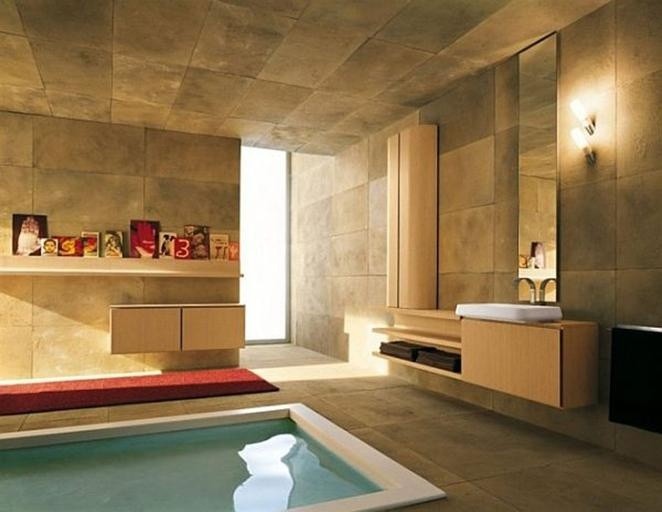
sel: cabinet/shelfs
[383,123,441,312]
[459,314,600,411]
[368,304,463,379]
[106,302,249,354]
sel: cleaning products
[531,241,546,269]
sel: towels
[377,338,436,362]
[607,326,661,434]
[415,348,461,373]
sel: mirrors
[514,28,564,304]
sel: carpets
[0,366,281,416]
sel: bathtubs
[0,401,448,512]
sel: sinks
[455,303,563,322]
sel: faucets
[514,277,536,305]
[538,277,557,302]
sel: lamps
[569,97,596,164]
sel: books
[12,214,238,260]
